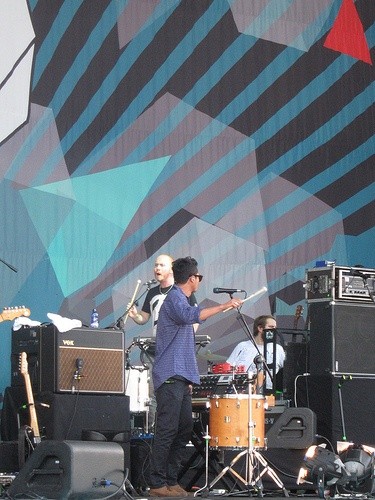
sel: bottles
[91,309,99,329]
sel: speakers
[288,375,375,453]
[308,300,375,377]
[0,322,132,499]
[261,406,317,450]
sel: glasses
[194,275,203,282]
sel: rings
[235,302,238,304]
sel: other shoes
[167,484,197,500]
[148,486,188,500]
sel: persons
[227,315,286,392]
[126,255,200,435]
[148,256,243,497]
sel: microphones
[77,358,83,369]
[143,279,158,285]
[213,287,245,294]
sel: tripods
[207,294,291,497]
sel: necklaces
[159,285,173,295]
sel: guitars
[283,305,306,376]
[0,305,31,323]
[18,351,41,445]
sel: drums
[206,394,269,452]
